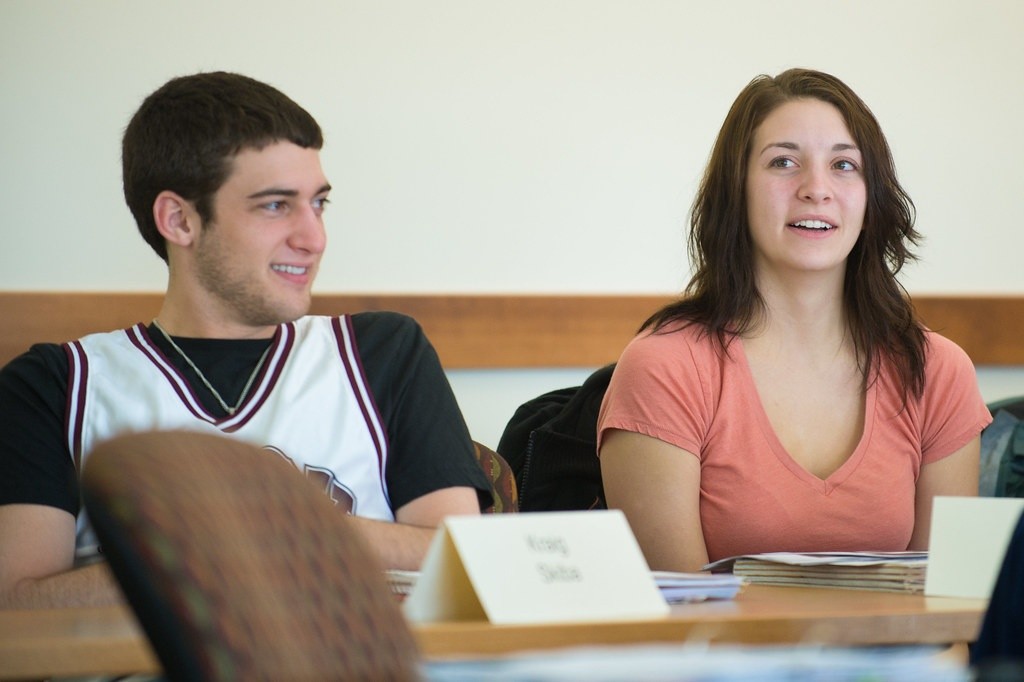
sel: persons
[0,71,494,611]
[594,65,995,574]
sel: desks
[0,583,991,682]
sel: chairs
[77,430,425,682]
[473,439,517,514]
